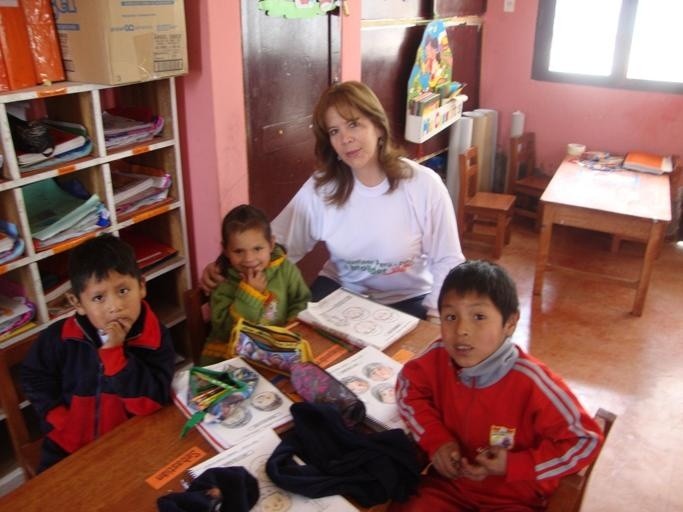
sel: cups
[568,143,586,162]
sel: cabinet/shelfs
[0,75,196,424]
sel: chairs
[548,406,618,512]
[457,145,517,261]
[0,332,65,478]
[510,131,552,232]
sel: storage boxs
[51,0,189,85]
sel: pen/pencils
[192,386,228,408]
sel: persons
[394,260,604,512]
[254,482,293,512]
[361,361,394,382]
[20,235,175,476]
[338,375,368,397]
[201,80,466,327]
[371,383,398,405]
[419,34,444,91]
[196,205,312,369]
[251,391,281,411]
[372,309,393,321]
[220,404,253,425]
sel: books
[297,287,419,351]
[410,81,462,118]
[103,111,163,150]
[0,222,24,264]
[623,150,674,175]
[21,179,109,252]
[1,284,37,344]
[170,357,296,454]
[324,345,411,436]
[182,426,361,512]
[36,259,75,315]
[119,229,178,272]
[110,164,171,216]
[16,116,92,174]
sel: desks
[532,152,672,318]
[0,291,446,512]
